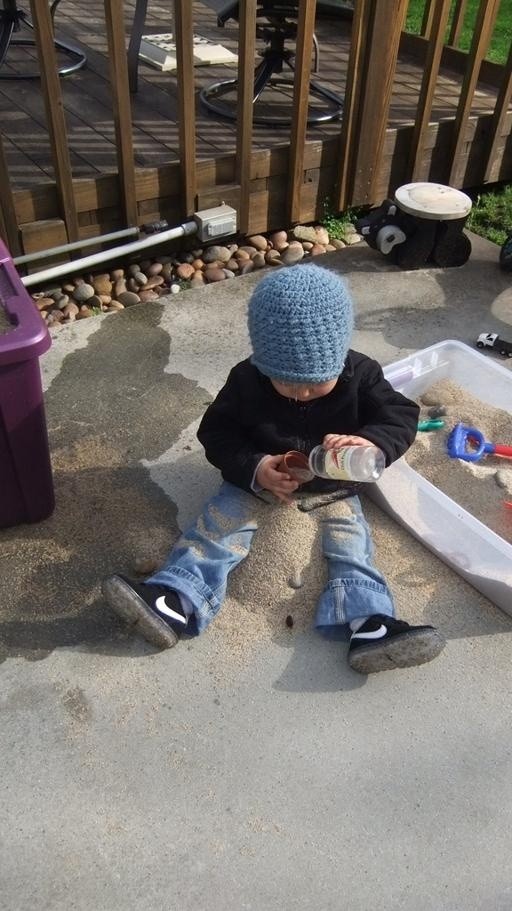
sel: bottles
[308,444,385,484]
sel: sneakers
[347,612,446,677]
[101,572,189,651]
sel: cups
[277,451,314,485]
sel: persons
[96,258,451,678]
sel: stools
[355,179,476,268]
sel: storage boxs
[0,238,56,531]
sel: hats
[244,261,354,385]
